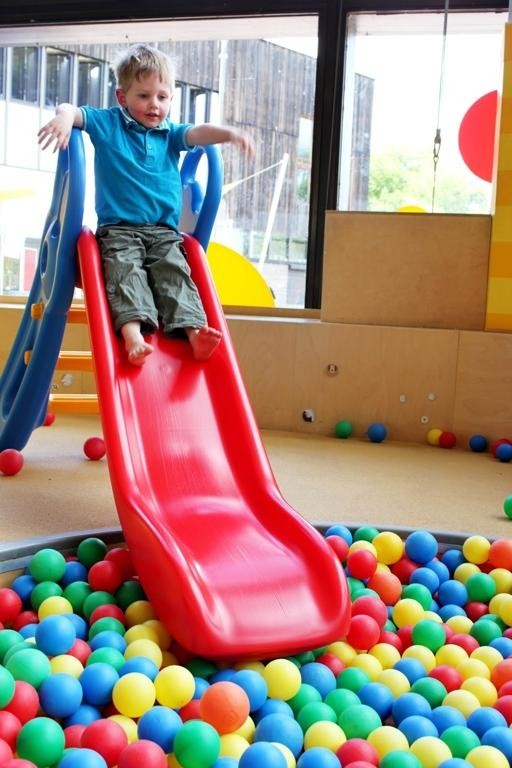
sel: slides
[76,229,351,662]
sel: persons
[36,44,254,366]
